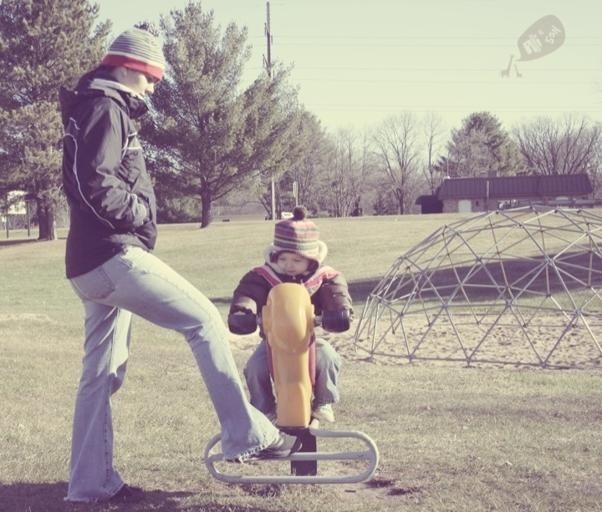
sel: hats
[270,205,320,272]
[102,28,164,80]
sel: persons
[229,206,354,427]
[60,28,302,504]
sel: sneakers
[312,404,334,422]
[106,484,145,501]
[229,430,303,461]
